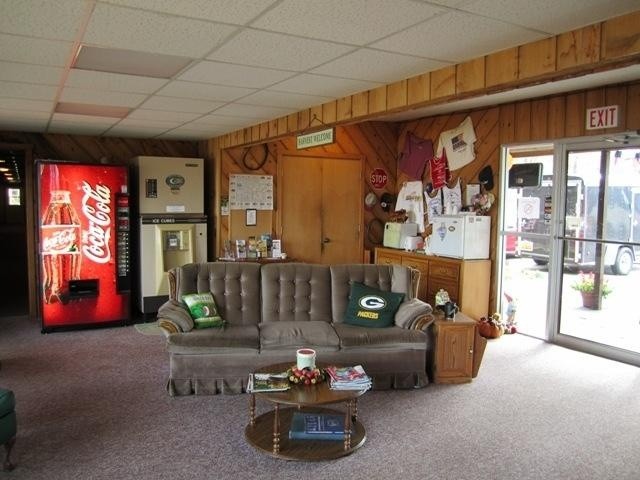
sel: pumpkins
[478,317,504,338]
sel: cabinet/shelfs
[432,313,475,386]
[374,248,491,319]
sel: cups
[295,348,317,375]
[444,301,460,320]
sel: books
[289,411,353,441]
[324,364,372,390]
[246,370,291,393]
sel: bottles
[40,181,82,305]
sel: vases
[581,291,599,307]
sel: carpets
[135,322,164,335]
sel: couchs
[160,262,428,396]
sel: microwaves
[430,215,490,260]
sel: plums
[290,366,325,384]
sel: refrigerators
[34,158,130,334]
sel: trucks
[514,174,640,275]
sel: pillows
[344,282,405,330]
[182,292,225,329]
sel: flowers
[572,269,613,294]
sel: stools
[0,384,18,470]
